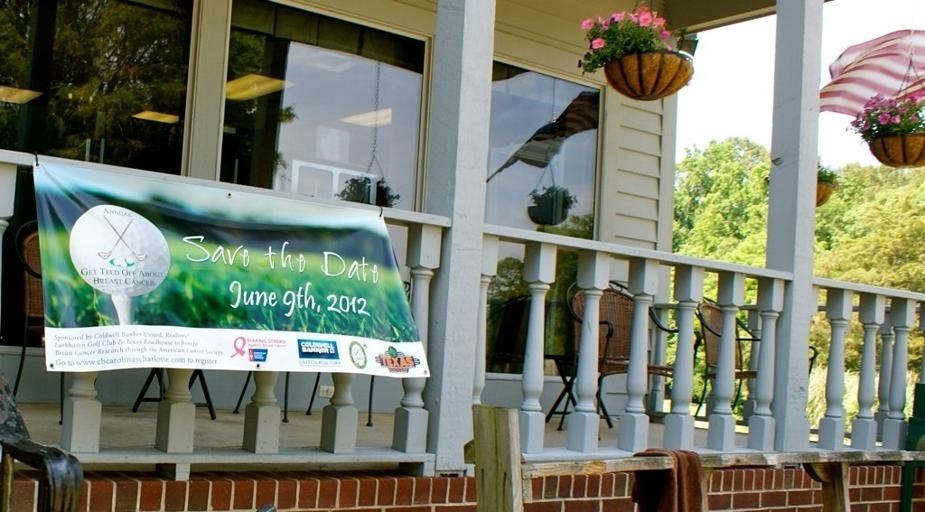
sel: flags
[489,89,599,178]
[817,26,925,115]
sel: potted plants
[816,166,840,207]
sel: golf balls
[69,204,171,296]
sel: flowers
[845,93,925,141]
[579,13,672,75]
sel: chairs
[691,296,818,428]
[487,293,549,373]
[14,221,375,427]
[547,279,705,433]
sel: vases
[604,49,694,101]
[868,133,925,169]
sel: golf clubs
[104,217,147,262]
[98,218,134,260]
